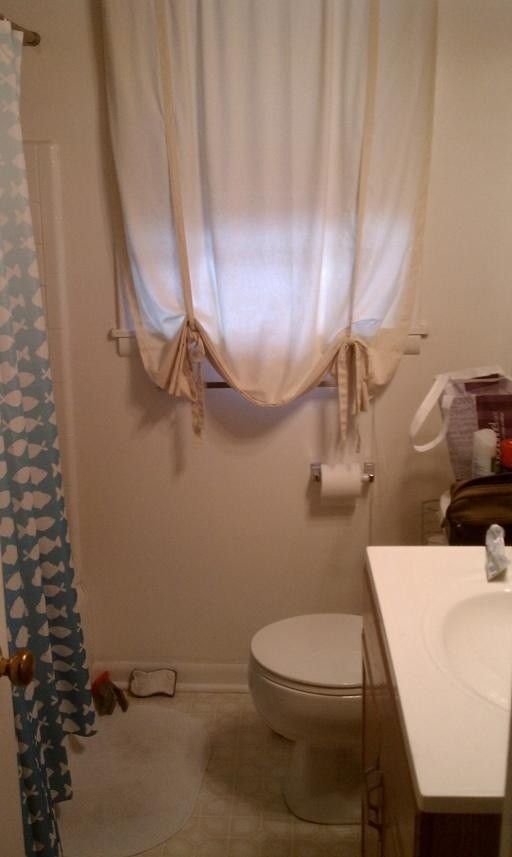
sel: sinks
[422,574,512,713]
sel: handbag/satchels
[407,365,512,546]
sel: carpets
[51,701,214,857]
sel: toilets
[249,614,363,826]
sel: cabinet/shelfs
[360,568,501,857]
